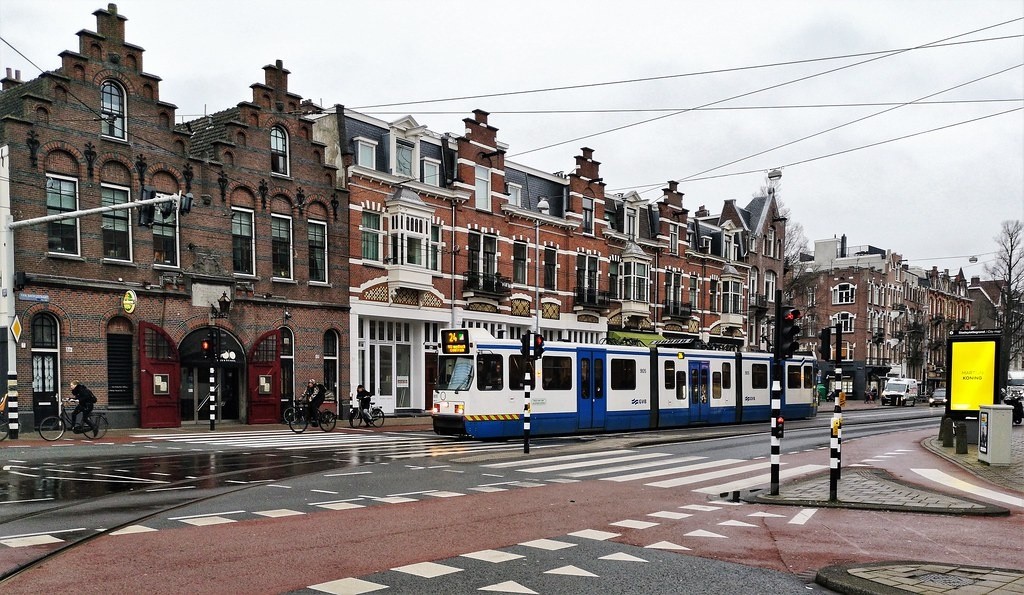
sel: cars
[929,387,947,407]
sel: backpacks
[81,384,98,403]
[316,384,326,396]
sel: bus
[431,327,819,439]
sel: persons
[496,360,503,379]
[1001,388,1009,405]
[68,380,99,437]
[356,385,374,425]
[864,385,877,404]
[299,379,327,420]
[478,362,484,385]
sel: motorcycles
[1000,388,1024,424]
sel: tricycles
[864,391,877,405]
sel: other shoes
[370,419,374,423]
[312,423,318,427]
[364,424,370,428]
[311,418,317,422]
[93,425,98,436]
[66,426,75,430]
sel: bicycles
[283,401,339,433]
[348,395,385,429]
[38,397,109,441]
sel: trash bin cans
[817,384,826,401]
[977,404,1015,467]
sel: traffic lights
[818,327,831,361]
[520,330,529,360]
[201,337,212,360]
[535,334,546,360]
[780,306,800,360]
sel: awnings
[609,331,667,348]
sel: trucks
[881,378,918,406]
